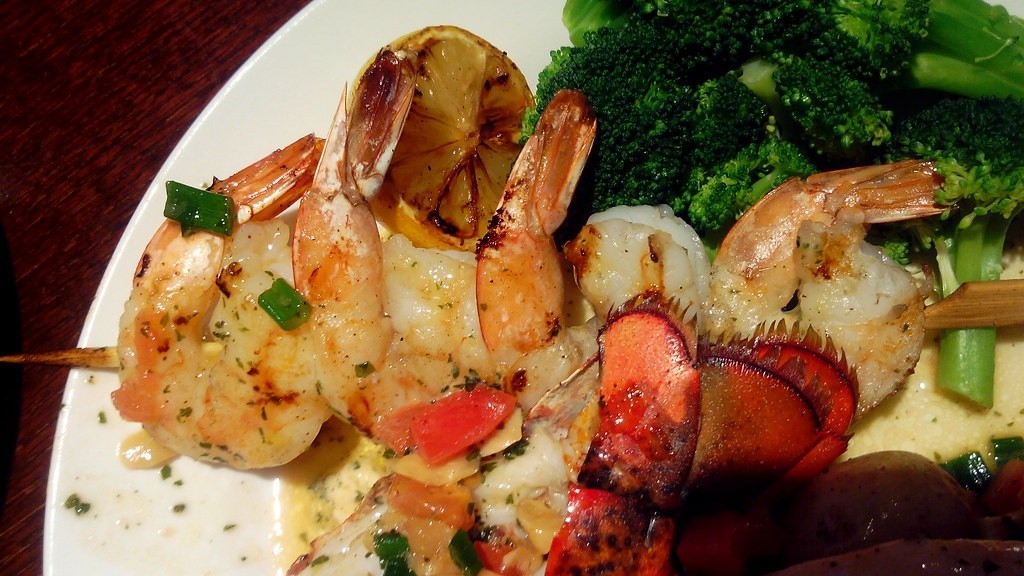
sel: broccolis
[522,1,1024,407]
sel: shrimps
[112,48,949,576]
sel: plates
[41,0,1024,576]
